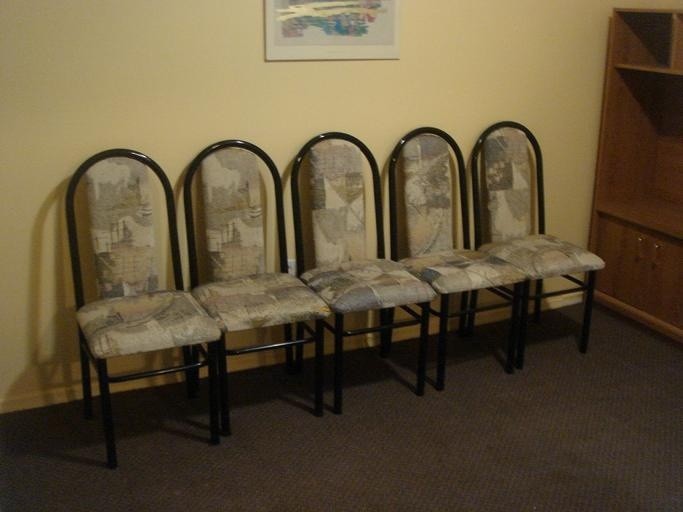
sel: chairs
[388,126,530,392]
[64,148,224,470]
[290,131,438,416]
[183,138,331,438]
[467,119,606,370]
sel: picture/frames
[264,0,401,61]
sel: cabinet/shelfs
[583,7,682,346]
